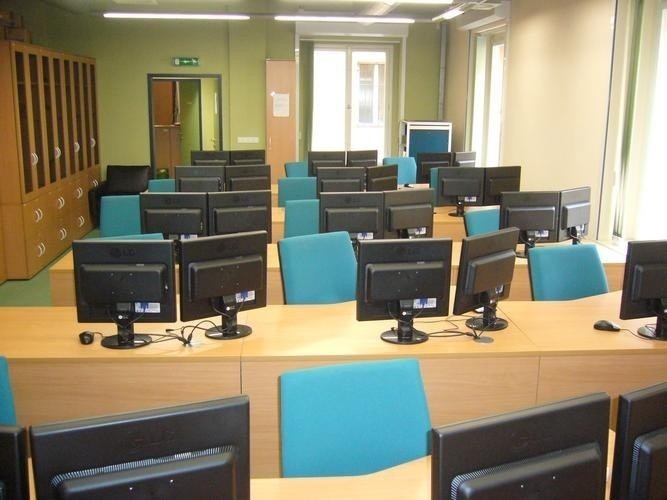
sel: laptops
[174,165,224,193]
[346,150,377,168]
[561,187,592,241]
[190,151,231,165]
[0,425,27,499]
[29,393,251,499]
[368,164,398,190]
[180,230,268,322]
[611,383,666,499]
[308,151,344,178]
[72,239,176,322]
[417,153,452,185]
[357,239,453,321]
[140,191,205,238]
[620,242,667,321]
[435,167,483,205]
[501,191,558,246]
[227,165,271,190]
[455,151,476,168]
[455,228,519,314]
[319,191,384,239]
[486,166,521,203]
[208,192,272,243]
[387,190,433,238]
[317,167,365,198]
[431,394,610,499]
[234,149,264,164]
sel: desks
[271,207,466,238]
[50,251,627,305]
[1,300,665,479]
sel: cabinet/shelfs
[0,41,101,284]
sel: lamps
[431,4,467,23]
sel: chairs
[464,210,500,236]
[276,358,435,477]
[101,196,142,237]
[277,230,360,304]
[284,198,320,236]
[285,162,311,178]
[279,178,317,207]
[526,243,608,299]
[148,178,176,193]
[383,157,418,184]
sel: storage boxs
[399,121,452,164]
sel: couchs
[88,165,151,227]
